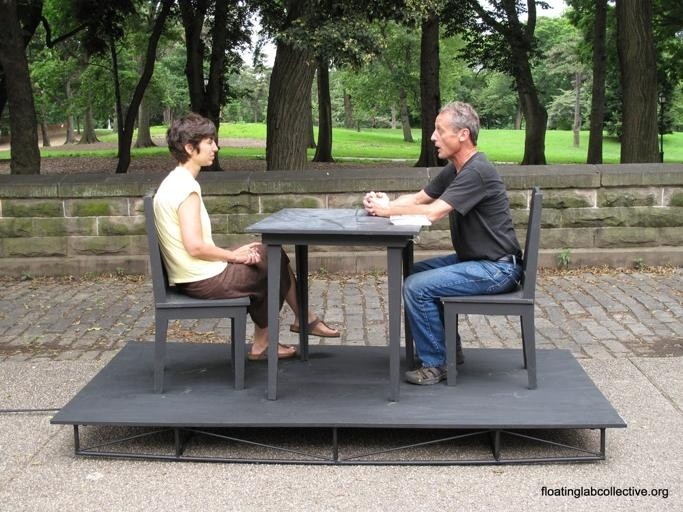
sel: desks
[241,204,426,405]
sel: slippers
[247,343,298,360]
[290,317,340,338]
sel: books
[390,212,432,227]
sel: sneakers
[405,345,465,386]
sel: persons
[151,112,341,361]
[362,101,525,387]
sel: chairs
[141,194,252,395]
[436,183,545,393]
